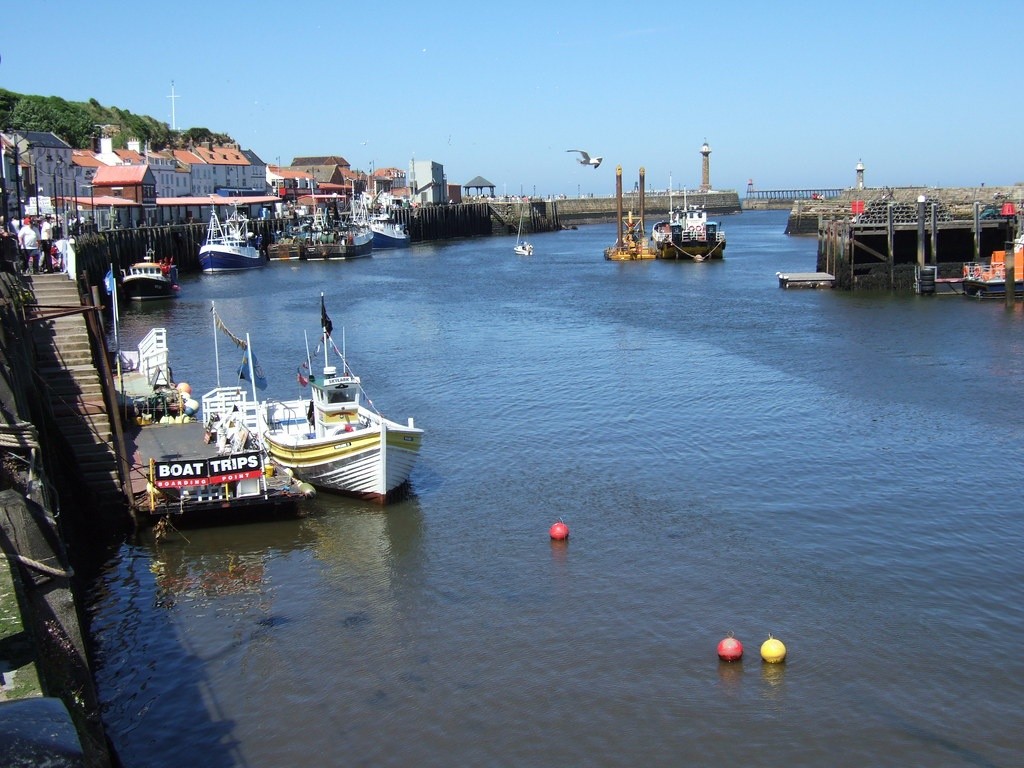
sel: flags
[298,369,309,386]
[103,271,114,294]
[321,299,333,337]
[237,347,267,392]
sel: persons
[9,214,95,274]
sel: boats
[198,203,268,275]
[259,292,426,510]
[268,206,374,261]
[348,183,410,252]
[961,237,1024,299]
[120,249,182,303]
[651,175,727,260]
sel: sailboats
[514,206,533,256]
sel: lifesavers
[920,269,936,293]
[696,225,702,231]
[689,225,695,231]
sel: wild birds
[564,148,604,170]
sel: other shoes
[33,271,40,275]
[44,271,55,274]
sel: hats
[45,214,54,220]
